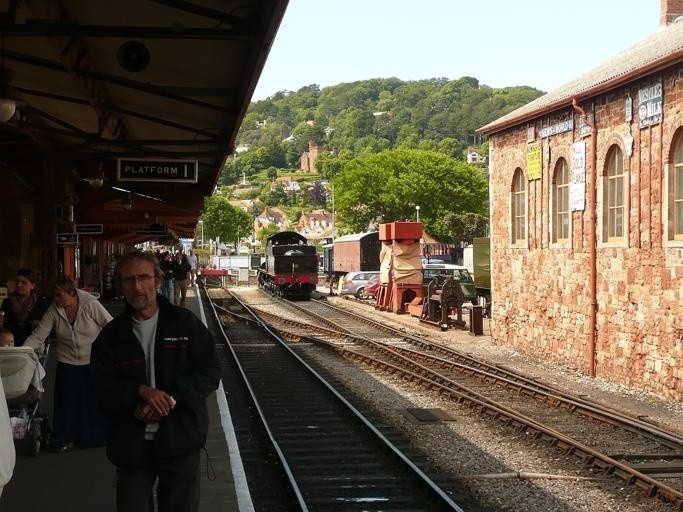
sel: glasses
[116,272,158,285]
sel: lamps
[80,143,113,189]
[0,26,29,123]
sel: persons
[20,272,115,453]
[0,270,50,417]
[86,248,197,308]
[0,330,15,348]
[90,249,221,511]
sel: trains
[258,230,319,300]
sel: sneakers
[48,438,70,454]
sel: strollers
[1,344,53,455]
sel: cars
[338,271,388,300]
